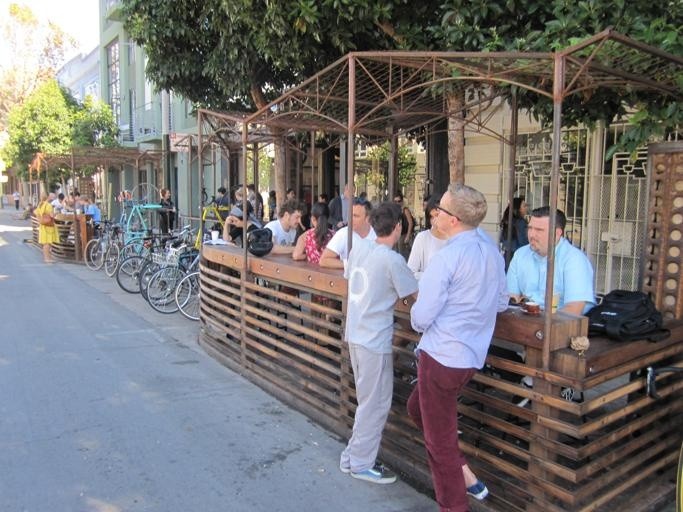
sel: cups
[75,209,80,215]
[211,231,219,241]
[551,295,559,313]
[525,302,538,315]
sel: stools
[255,283,305,353]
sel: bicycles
[83,181,233,322]
[402,215,426,253]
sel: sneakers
[349,464,398,484]
[465,478,488,500]
[339,458,385,473]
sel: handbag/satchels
[40,204,54,226]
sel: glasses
[432,201,463,223]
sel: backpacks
[583,289,670,343]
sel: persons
[13,183,101,262]
[157,189,176,251]
